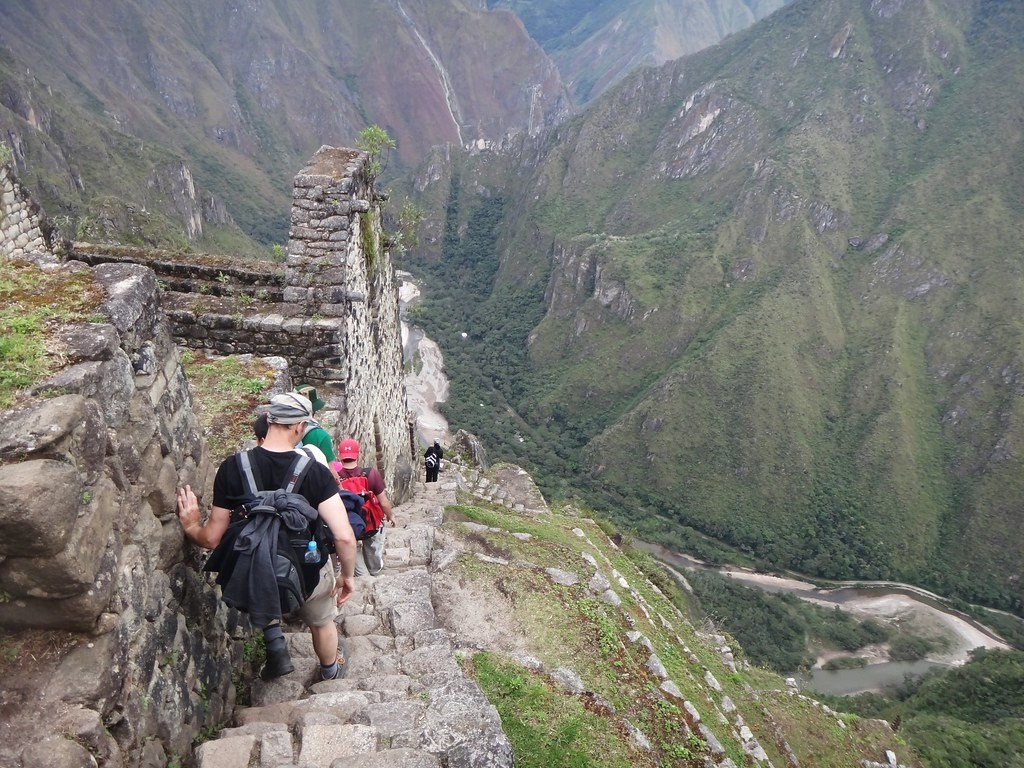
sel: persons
[424,438,443,482]
[177,392,356,680]
[254,384,396,577]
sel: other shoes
[320,645,348,682]
[258,638,294,682]
[370,558,384,577]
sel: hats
[339,437,359,461]
[434,438,439,444]
[293,386,325,412]
[267,393,319,426]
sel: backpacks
[424,452,437,468]
[214,451,315,620]
[338,467,383,539]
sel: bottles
[304,540,321,563]
